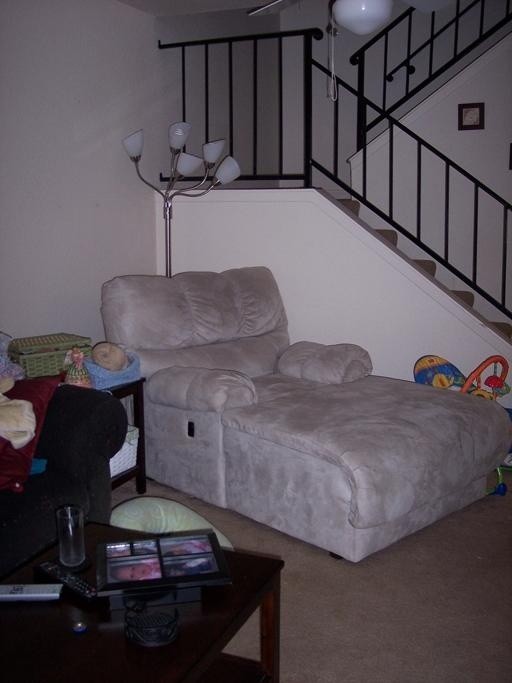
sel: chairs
[1,377,128,576]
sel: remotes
[39,561,96,598]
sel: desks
[0,519,282,682]
[84,375,148,497]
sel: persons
[160,536,212,560]
[110,559,161,580]
[64,342,91,387]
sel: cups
[57,506,87,567]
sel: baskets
[7,332,92,377]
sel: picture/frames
[455,102,484,131]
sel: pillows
[110,493,235,550]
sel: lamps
[122,118,240,277]
[328,0,397,37]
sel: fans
[246,0,456,100]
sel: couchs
[90,264,512,564]
